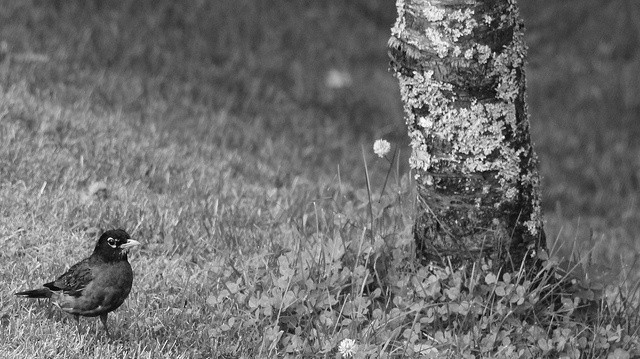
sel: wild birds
[13,227,142,345]
